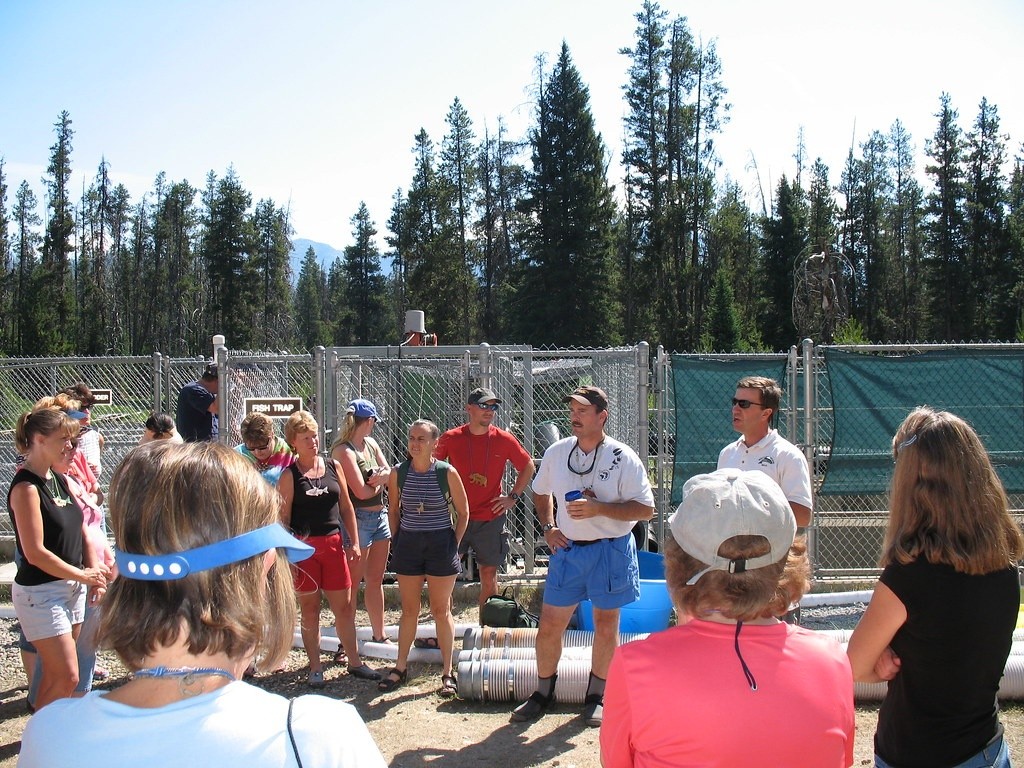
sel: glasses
[70,438,79,451]
[567,437,605,474]
[478,402,499,410]
[80,402,92,413]
[244,439,269,451]
[372,416,378,423]
[730,397,765,409]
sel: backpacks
[481,585,539,628]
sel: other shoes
[93,668,110,679]
[348,664,381,679]
[511,691,557,722]
[307,668,324,689]
[585,695,603,727]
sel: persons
[225,384,655,729]
[13,439,389,768]
[138,412,184,444]
[846,403,1024,768]
[599,466,856,768]
[7,382,116,712]
[716,376,814,627]
[176,362,218,441]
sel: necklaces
[24,460,73,507]
[132,665,237,699]
[39,473,55,496]
[412,458,434,515]
[296,456,329,496]
[576,441,605,500]
[255,451,274,471]
[467,421,489,488]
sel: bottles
[564,490,583,517]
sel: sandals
[333,644,348,665]
[414,637,439,649]
[372,636,392,643]
[440,672,458,696]
[376,668,408,692]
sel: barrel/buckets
[577,550,673,633]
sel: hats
[668,468,798,585]
[113,521,316,581]
[349,399,381,422]
[67,411,87,419]
[468,387,502,405]
[561,386,608,411]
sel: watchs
[542,523,556,533]
[508,491,522,502]
